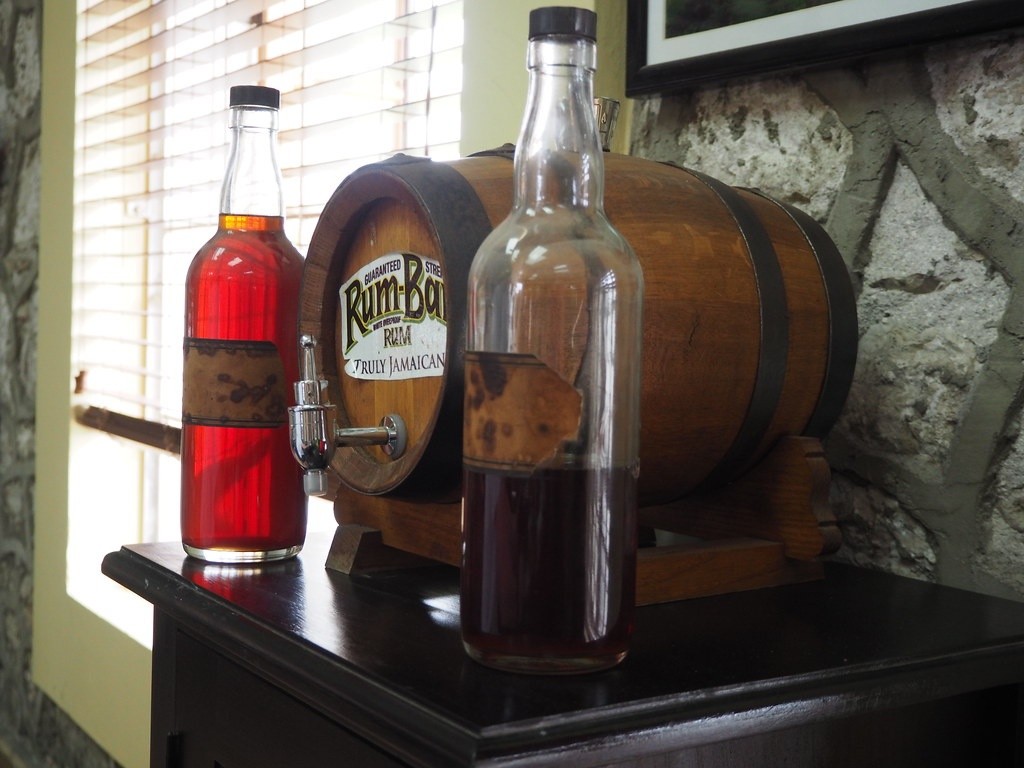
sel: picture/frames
[625,0,1024,105]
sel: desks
[101,524,1024,768]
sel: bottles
[181,85,307,566]
[461,6,645,670]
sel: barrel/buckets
[294,145,858,497]
